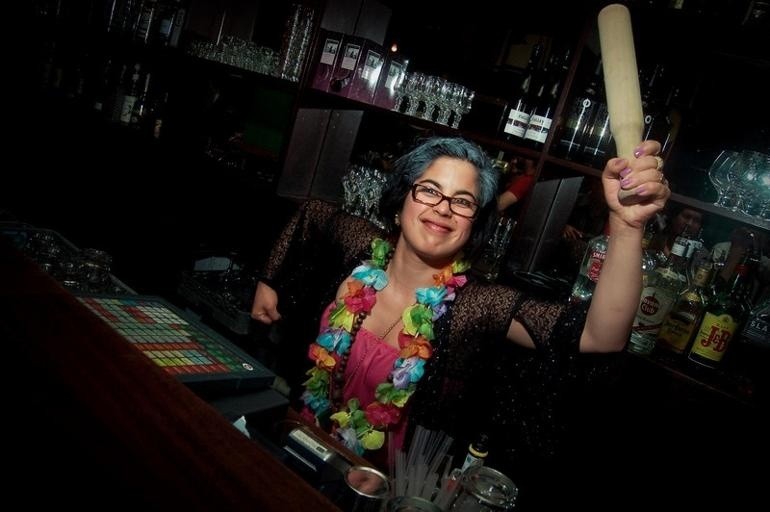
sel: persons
[494,156,535,221]
[563,190,606,241]
[649,208,712,278]
[248,136,674,493]
[706,225,769,297]
[342,133,400,231]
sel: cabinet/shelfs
[274,3,590,309]
[520,0,770,420]
[2,1,280,264]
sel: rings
[658,172,666,184]
[654,156,664,171]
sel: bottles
[566,215,770,374]
[458,435,492,478]
[90,0,187,144]
[560,42,679,182]
[494,39,577,147]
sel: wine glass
[386,65,476,130]
[336,159,388,220]
[705,148,770,229]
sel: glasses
[408,183,485,219]
